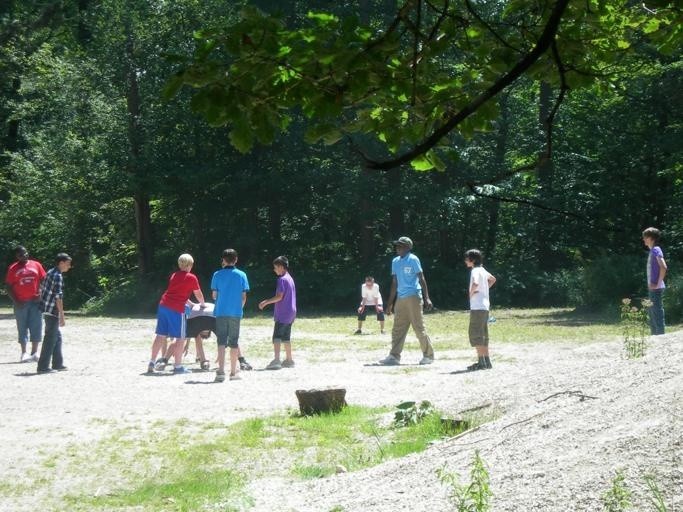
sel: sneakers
[37,368,57,373]
[380,355,400,364]
[148,358,251,381]
[420,355,434,364]
[486,363,492,368]
[281,360,294,368]
[21,352,31,361]
[52,366,67,371]
[266,360,280,369]
[467,364,487,370]
[30,352,40,361]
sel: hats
[394,237,413,248]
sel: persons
[210,248,252,383]
[379,235,435,365]
[641,226,668,337]
[147,252,207,375]
[37,252,73,375]
[463,248,497,371]
[157,301,254,371]
[353,275,386,335]
[4,246,48,364]
[258,256,298,370]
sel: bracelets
[424,295,431,299]
